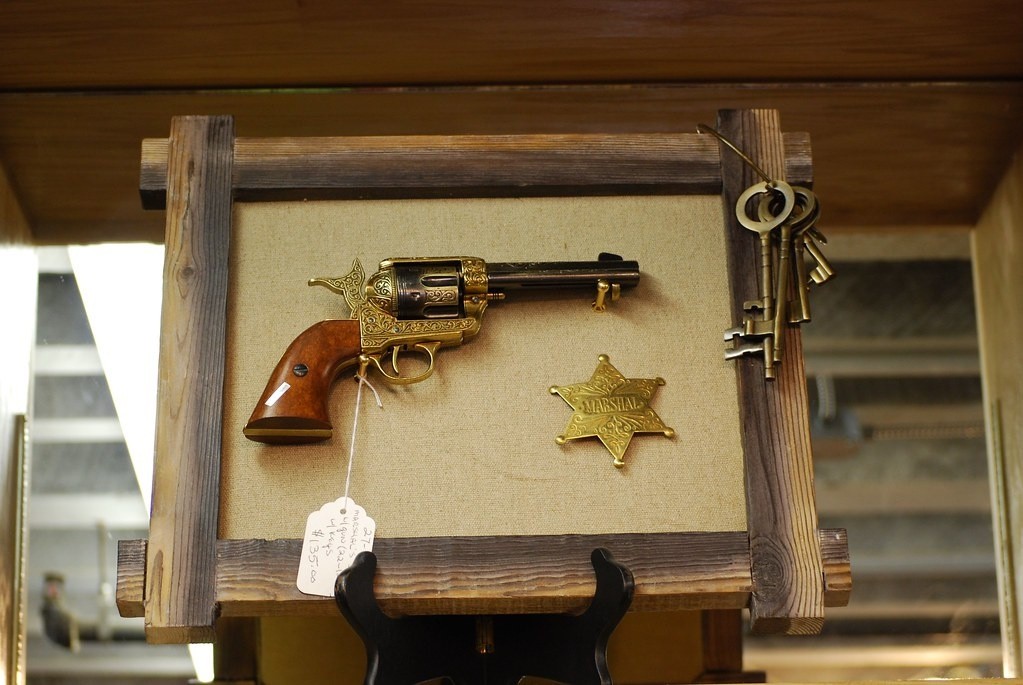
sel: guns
[244,252,640,443]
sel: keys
[725,179,837,381]
